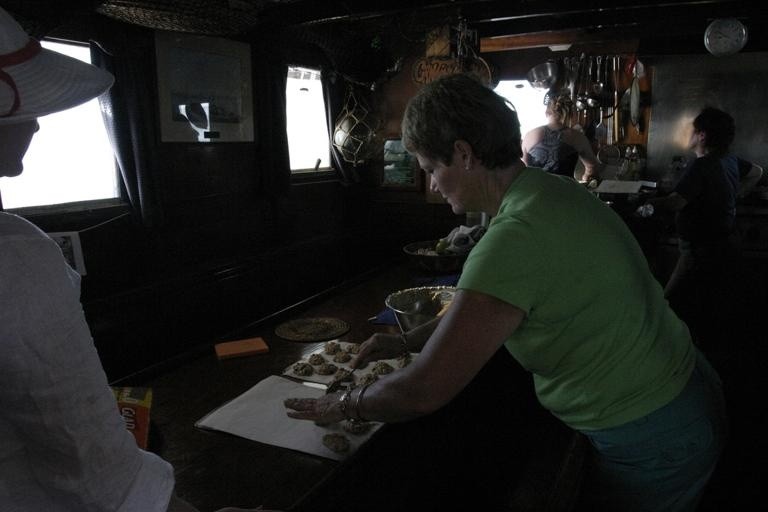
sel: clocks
[703,16,748,57]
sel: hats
[0,7,118,129]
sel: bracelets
[356,373,378,428]
[336,376,356,429]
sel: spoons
[325,364,358,394]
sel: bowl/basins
[384,286,458,352]
[403,241,459,275]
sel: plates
[275,317,350,342]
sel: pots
[525,60,560,88]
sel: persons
[284,74,732,512]
[1,9,282,512]
[527,90,599,184]
[657,106,764,310]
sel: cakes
[292,340,394,453]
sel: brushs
[595,107,607,140]
